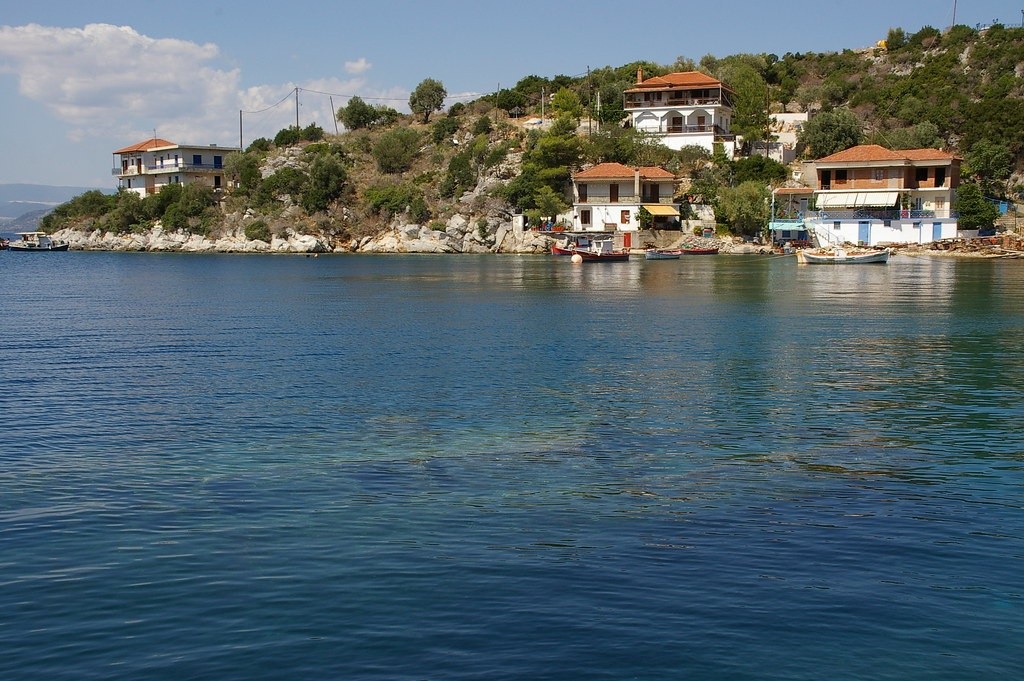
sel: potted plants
[552,222,566,231]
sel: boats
[550,242,574,256]
[678,247,721,254]
[801,245,889,263]
[7,231,70,252]
[645,247,682,260]
[572,234,630,262]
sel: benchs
[604,223,618,230]
